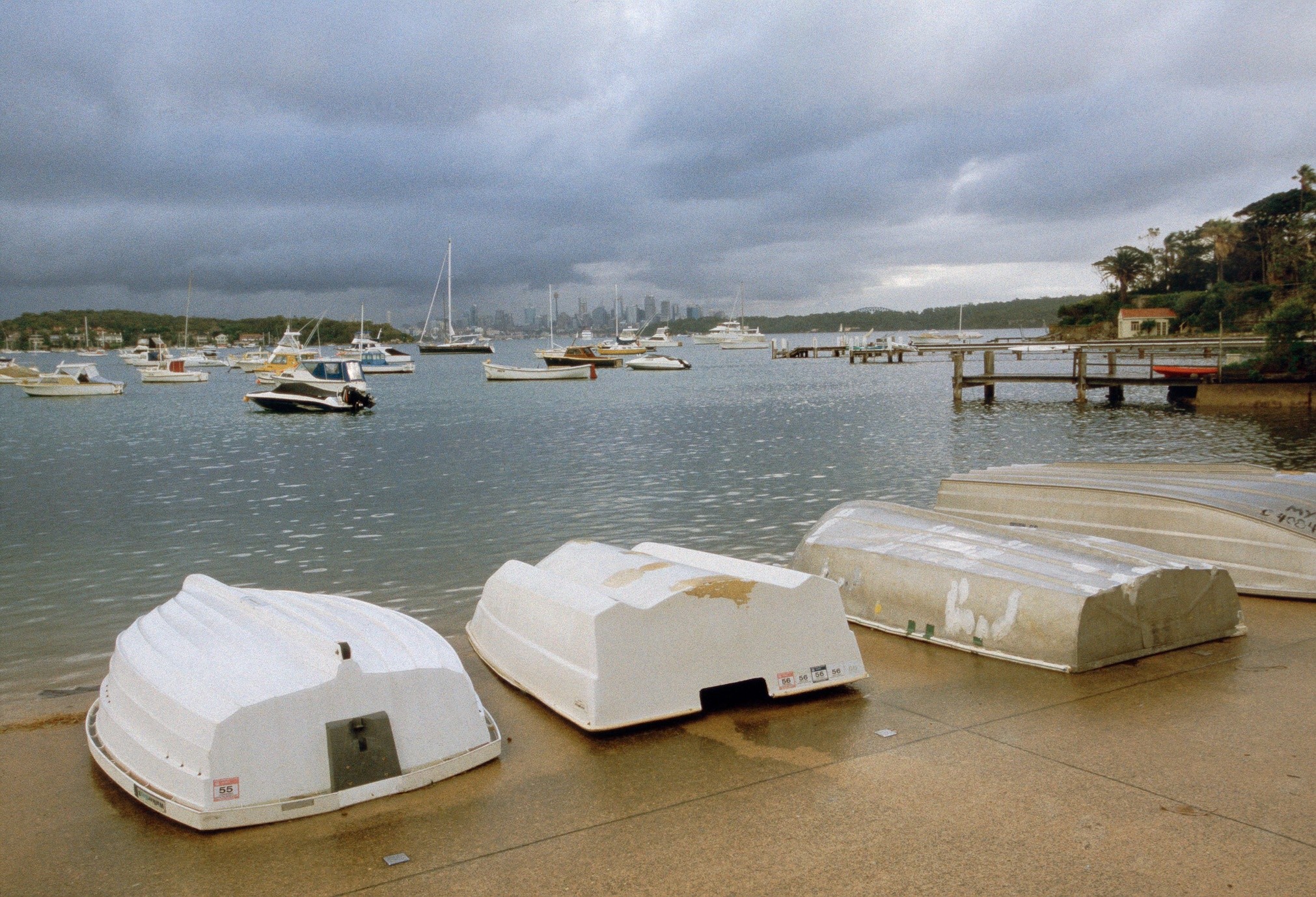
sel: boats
[243,381,377,414]
[782,498,1248,674]
[1151,363,1217,376]
[542,332,624,368]
[852,302,986,352]
[0,272,417,384]
[269,323,368,397]
[482,358,598,381]
[594,277,772,355]
[581,330,594,341]
[83,572,504,833]
[934,459,1316,601]
[464,536,871,734]
[624,352,693,370]
[15,361,127,397]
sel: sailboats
[532,284,567,359]
[413,238,496,354]
